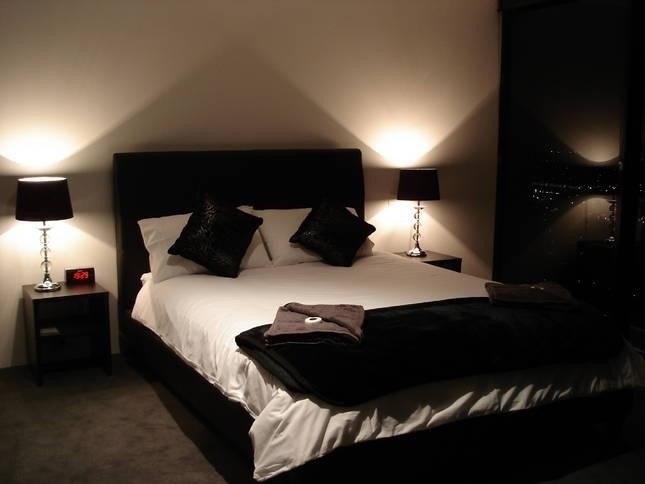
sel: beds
[114,148,633,484]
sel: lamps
[397,168,440,257]
[17,177,74,292]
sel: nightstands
[22,281,112,386]
[394,251,462,272]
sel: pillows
[168,190,263,277]
[290,192,375,267]
[138,205,272,282]
[255,207,374,266]
